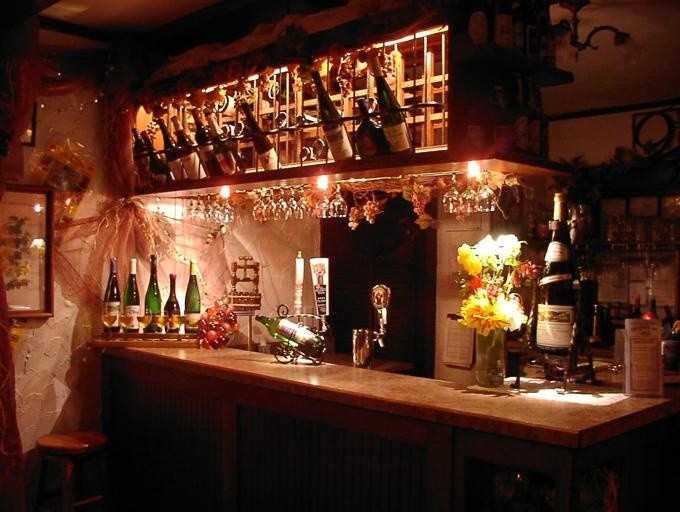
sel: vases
[474,324,506,387]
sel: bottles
[253,313,327,354]
[128,95,280,186]
[309,55,415,162]
[631,293,674,334]
[536,191,576,354]
[103,255,204,334]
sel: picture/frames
[0,183,55,322]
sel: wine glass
[185,196,234,226]
[444,171,496,213]
[252,185,350,222]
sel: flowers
[454,234,526,332]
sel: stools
[34,431,110,512]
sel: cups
[352,328,374,368]
